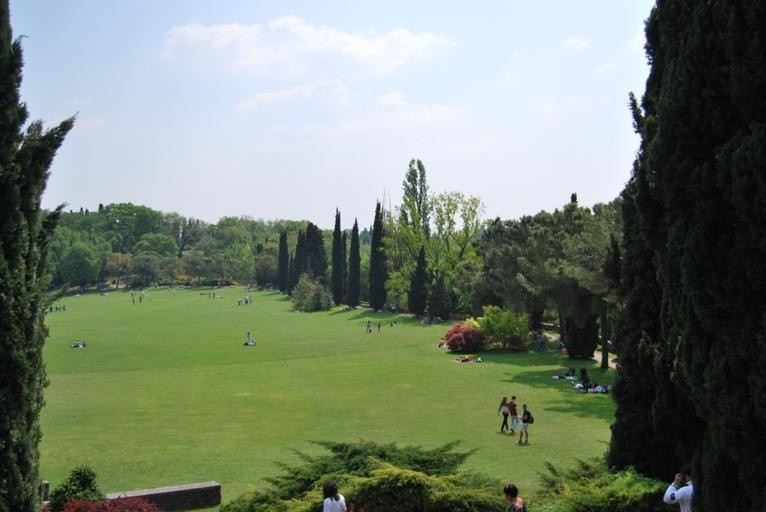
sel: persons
[321,479,347,512]
[662,461,696,512]
[497,396,509,432]
[504,396,519,433]
[41,281,273,348]
[549,365,621,395]
[364,300,569,354]
[503,483,527,512]
[515,404,535,444]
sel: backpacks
[529,415,534,424]
